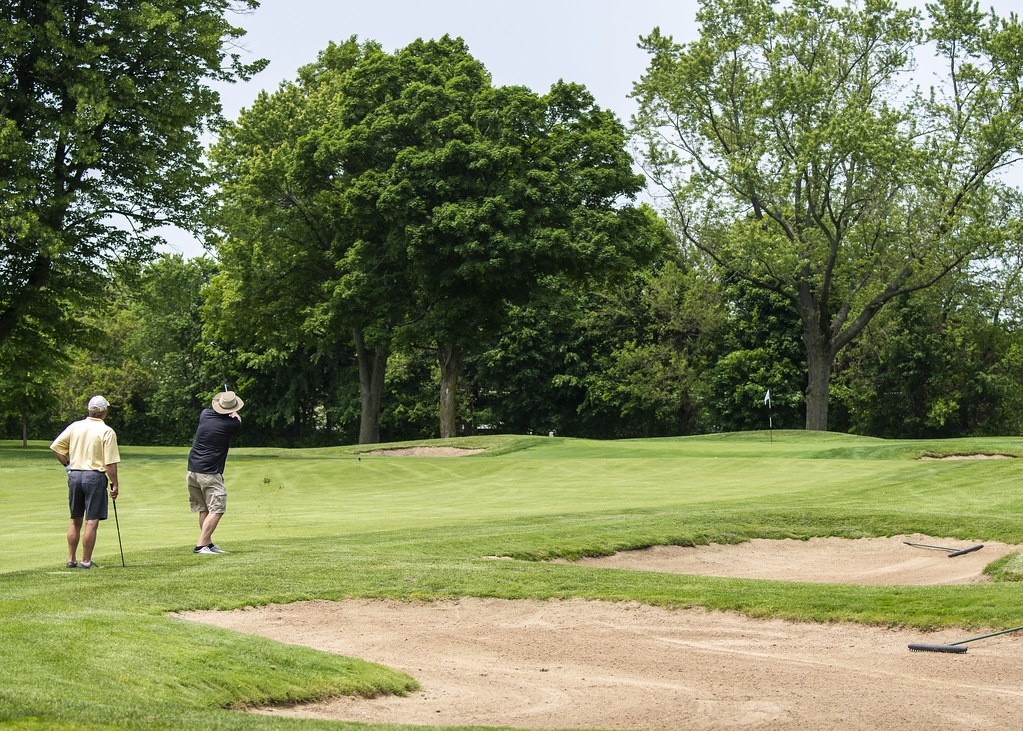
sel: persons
[50,395,120,569]
[186,390,244,555]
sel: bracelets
[64,461,70,467]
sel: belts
[71,470,99,472]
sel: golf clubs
[109,482,125,567]
[210,341,228,392]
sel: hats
[87,395,111,413]
[211,390,245,414]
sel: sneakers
[78,561,99,568]
[66,561,80,569]
[206,543,227,553]
[194,546,220,554]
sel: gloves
[66,465,70,473]
